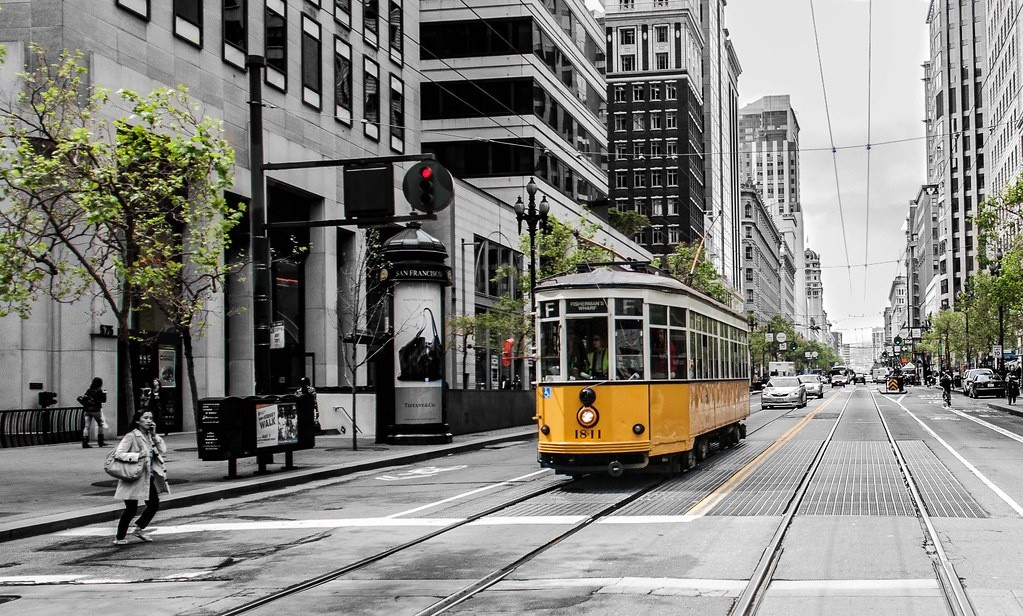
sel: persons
[1005,363,1022,405]
[585,331,608,380]
[295,377,319,420]
[145,378,168,436]
[82,377,109,448]
[940,371,952,407]
[652,329,677,378]
[114,409,170,544]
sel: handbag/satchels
[104,431,146,481]
[314,419,321,435]
[77,394,94,408]
[398,307,440,382]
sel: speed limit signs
[992,345,1002,358]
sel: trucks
[769,362,797,377]
[872,369,885,381]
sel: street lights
[989,247,1005,377]
[958,289,974,370]
[514,176,552,385]
[462,242,480,388]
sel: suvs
[854,373,865,385]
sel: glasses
[593,337,599,341]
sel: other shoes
[1013,402,1016,404]
[113,538,130,545]
[133,529,152,542]
[1006,403,1010,405]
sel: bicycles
[942,390,950,407]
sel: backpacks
[942,375,951,389]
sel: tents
[901,362,917,372]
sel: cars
[797,375,824,398]
[864,375,873,383]
[902,374,913,384]
[963,369,1005,398]
[876,376,886,384]
[761,376,807,408]
[831,375,845,387]
[820,376,830,384]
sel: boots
[82,436,93,449]
[97,434,108,448]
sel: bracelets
[316,412,319,413]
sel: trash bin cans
[197,394,316,480]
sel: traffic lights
[896,337,900,344]
[419,163,436,213]
[793,342,795,350]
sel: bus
[811,369,826,375]
[831,366,854,383]
[533,263,751,481]
